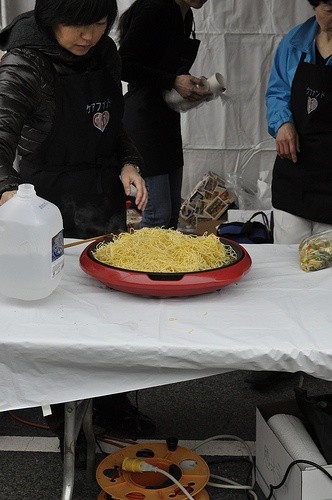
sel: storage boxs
[255,394,332,500]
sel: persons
[0,0,149,241]
[115,0,214,231]
[264,0,332,247]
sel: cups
[165,73,227,112]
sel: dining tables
[0,237,332,500]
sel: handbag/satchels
[216,211,273,245]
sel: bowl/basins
[79,231,253,297]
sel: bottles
[0,184,64,300]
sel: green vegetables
[304,240,332,266]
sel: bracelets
[124,163,142,176]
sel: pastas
[91,225,238,273]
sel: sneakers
[90,393,155,435]
[56,425,87,467]
